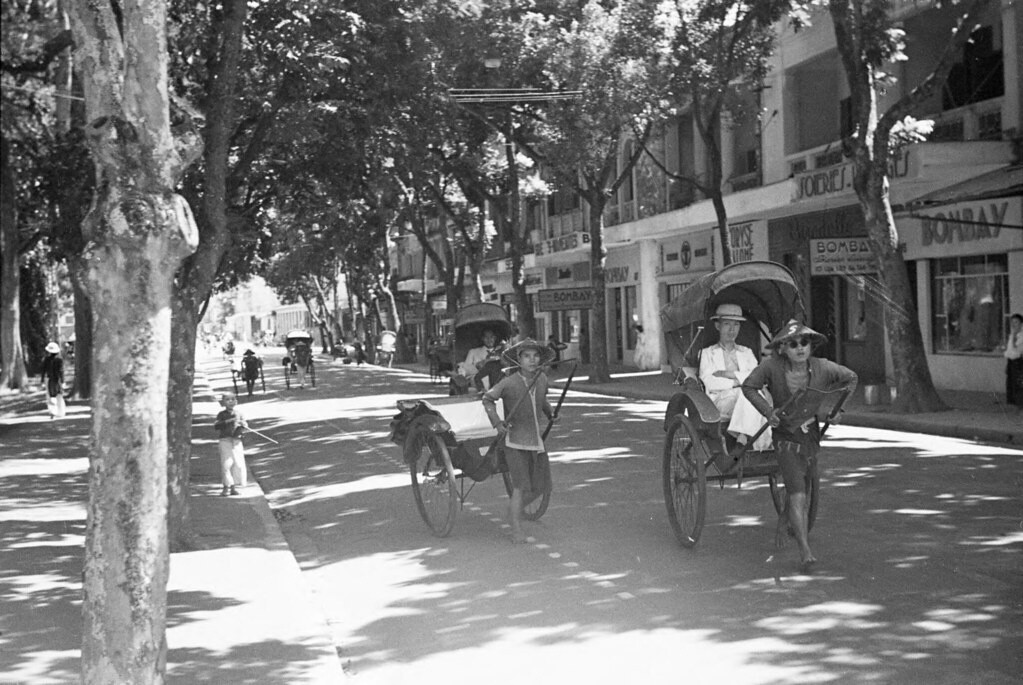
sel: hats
[764,318,827,349]
[502,337,556,369]
[710,304,747,321]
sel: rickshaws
[230,357,266,398]
[281,329,316,390]
[447,303,516,401]
[659,260,852,550]
[428,335,450,385]
[387,355,579,539]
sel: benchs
[397,395,505,448]
[677,366,773,455]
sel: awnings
[908,163,1023,230]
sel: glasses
[790,338,808,348]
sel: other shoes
[729,442,746,455]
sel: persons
[40,342,67,419]
[742,319,858,567]
[463,329,560,540]
[351,327,417,365]
[242,349,258,396]
[696,304,775,451]
[295,342,307,388]
[213,391,247,495]
[547,334,559,370]
[1005,314,1023,410]
